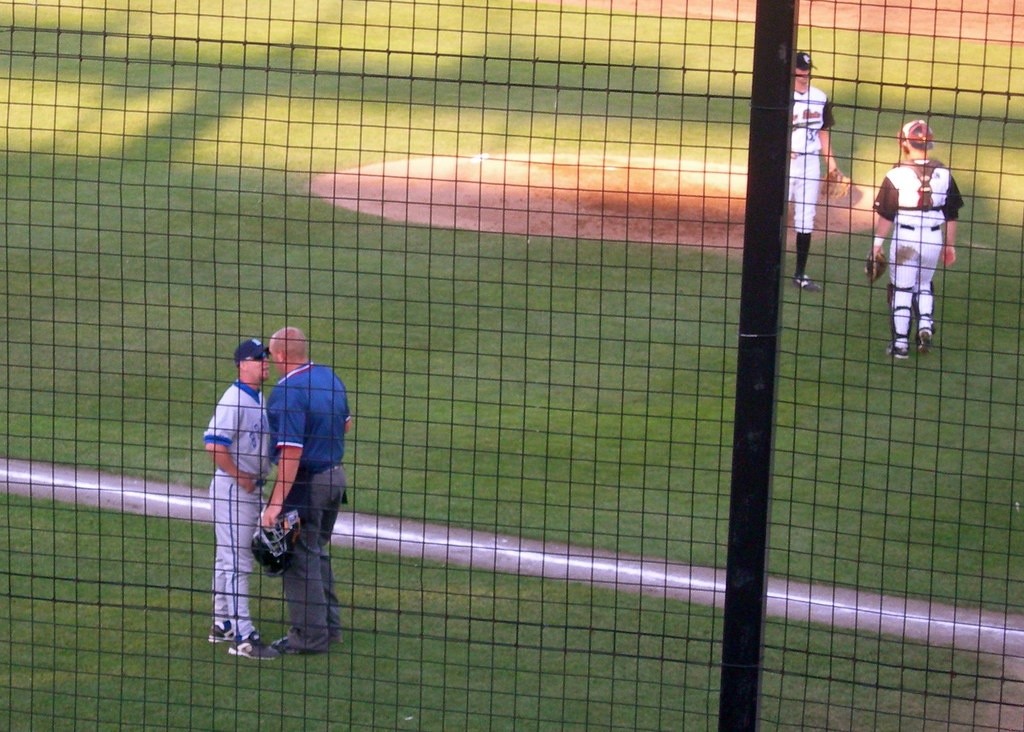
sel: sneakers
[228,632,282,659]
[208,619,235,644]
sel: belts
[901,224,940,231]
[317,465,342,472]
[255,478,267,486]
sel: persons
[787,52,851,293]
[869,120,965,359]
[204,339,280,660]
[265,328,351,656]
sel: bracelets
[874,237,885,246]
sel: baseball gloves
[820,169,852,201]
[864,250,887,283]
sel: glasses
[247,351,269,361]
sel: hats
[903,119,934,150]
[795,52,817,71]
[235,340,271,366]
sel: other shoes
[792,273,821,292]
[886,345,908,359]
[270,636,321,653]
[917,330,932,353]
